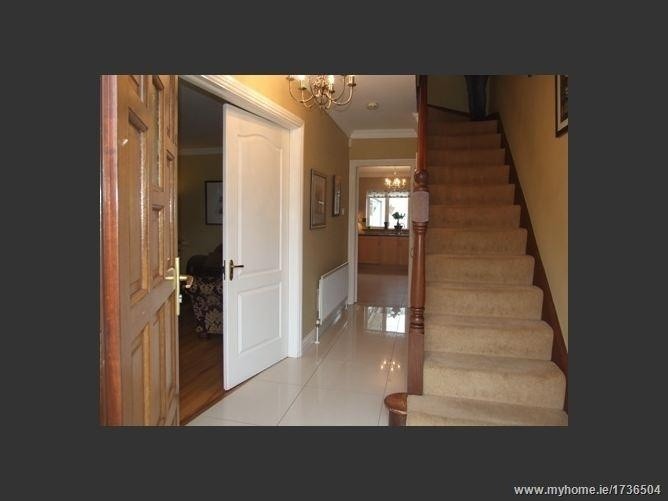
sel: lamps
[286,76,357,111]
[383,170,407,191]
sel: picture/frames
[556,75,569,137]
[332,174,341,216]
[310,169,327,230]
[204,181,222,226]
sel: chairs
[184,244,223,338]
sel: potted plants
[393,212,406,230]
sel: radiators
[314,262,349,345]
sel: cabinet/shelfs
[358,234,397,265]
[397,236,408,266]
